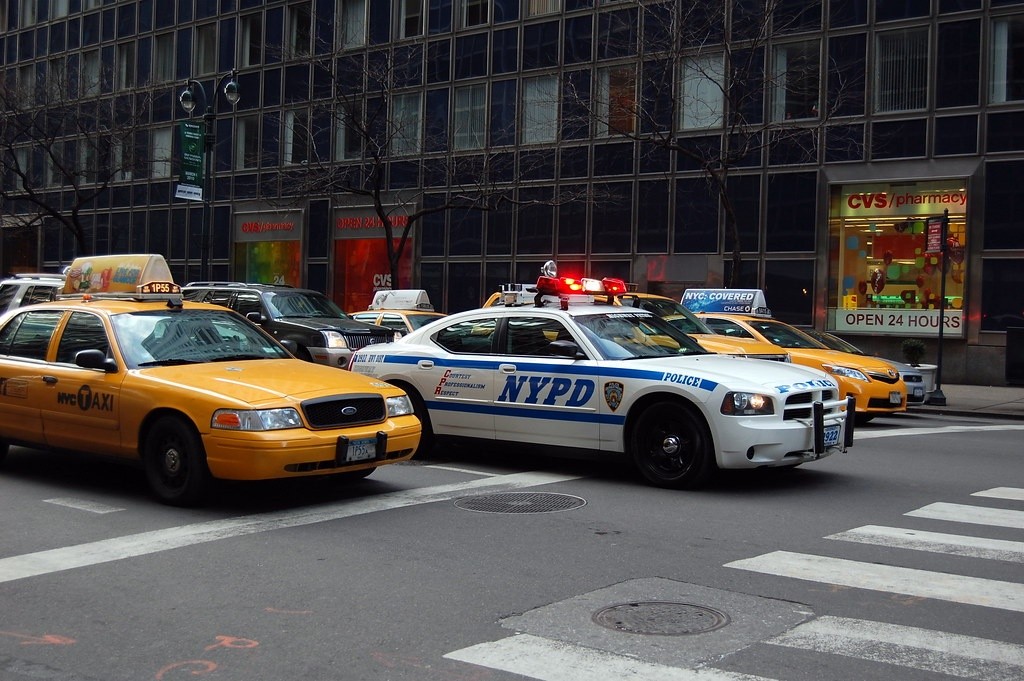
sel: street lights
[178,69,242,280]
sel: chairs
[556,328,574,343]
[512,328,550,356]
[439,329,513,353]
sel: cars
[0,252,932,449]
[346,277,857,490]
[0,280,423,509]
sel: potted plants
[901,339,937,392]
